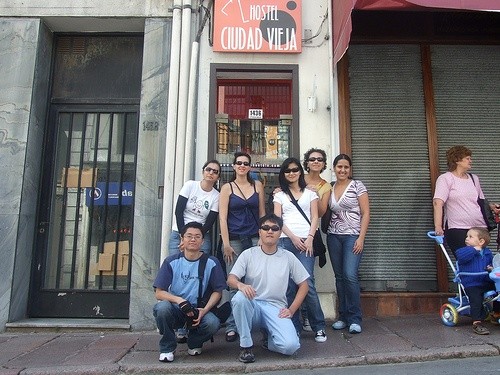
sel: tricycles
[426,231,500,328]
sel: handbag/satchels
[313,228,326,268]
[321,182,336,234]
[468,173,498,231]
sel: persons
[433,145,496,258]
[273,158,328,343]
[153,221,228,362]
[455,227,500,334]
[493,203,500,253]
[226,214,310,362]
[219,153,266,342]
[168,160,221,343]
[273,147,332,331]
[327,153,370,334]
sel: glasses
[285,167,301,173]
[235,161,250,166]
[307,157,324,162]
[203,167,220,174]
[260,225,280,231]
[183,235,201,240]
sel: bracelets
[309,234,314,238]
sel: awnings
[85,182,134,214]
[332,0,500,64]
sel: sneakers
[177,330,188,342]
[238,345,255,363]
[349,323,361,333]
[260,328,268,349]
[315,329,327,343]
[473,322,490,335]
[187,347,201,356]
[490,312,500,320]
[303,319,312,331]
[331,321,347,330]
[159,350,176,362]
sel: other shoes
[226,331,237,342]
